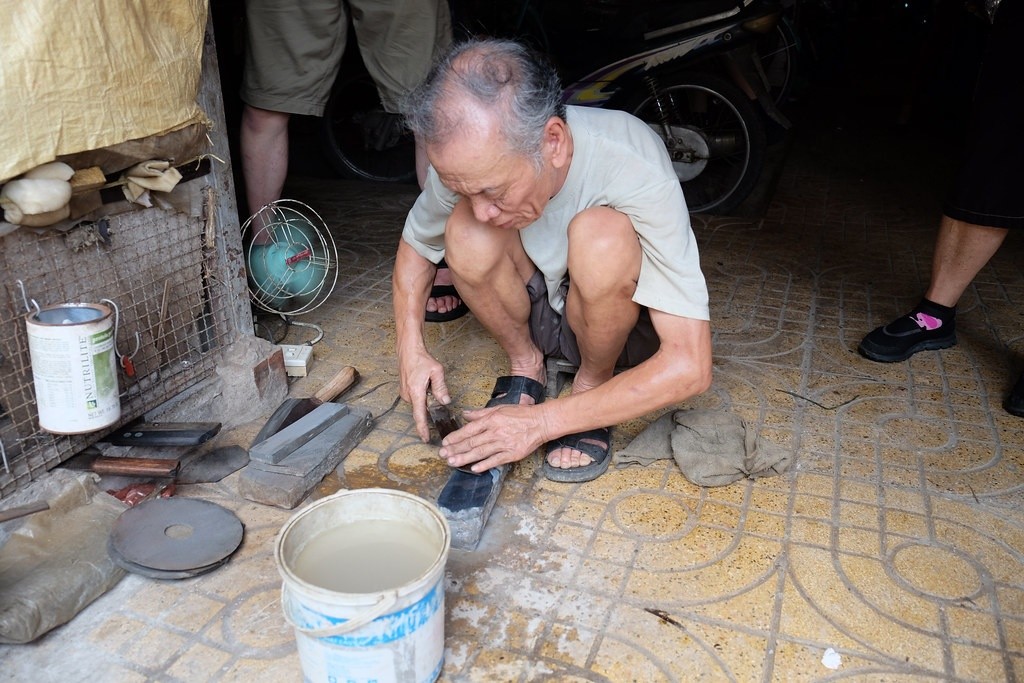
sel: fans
[240,198,339,346]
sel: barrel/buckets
[274,488,452,683]
[24,301,121,435]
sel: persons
[390,39,713,483]
[240,0,472,322]
[857,0,1024,418]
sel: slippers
[485,374,546,408]
[542,427,612,482]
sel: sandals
[424,261,469,322]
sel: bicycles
[321,0,797,215]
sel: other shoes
[1005,371,1024,417]
[857,304,957,362]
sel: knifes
[49,454,182,478]
[426,388,482,476]
[246,364,360,456]
